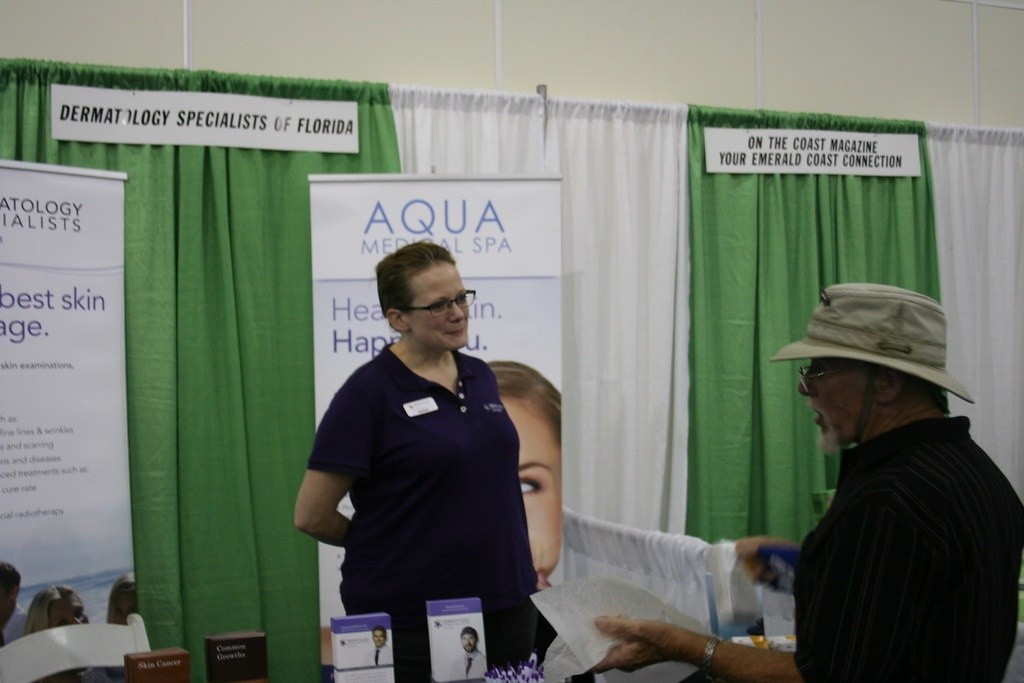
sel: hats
[768,283,976,445]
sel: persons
[592,283,1024,683]
[0,561,137,683]
[451,626,486,678]
[295,243,540,683]
[486,362,562,592]
[363,625,393,665]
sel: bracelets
[701,635,719,674]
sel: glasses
[401,289,476,319]
[798,365,863,397]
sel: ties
[374,649,380,668]
[466,657,473,679]
